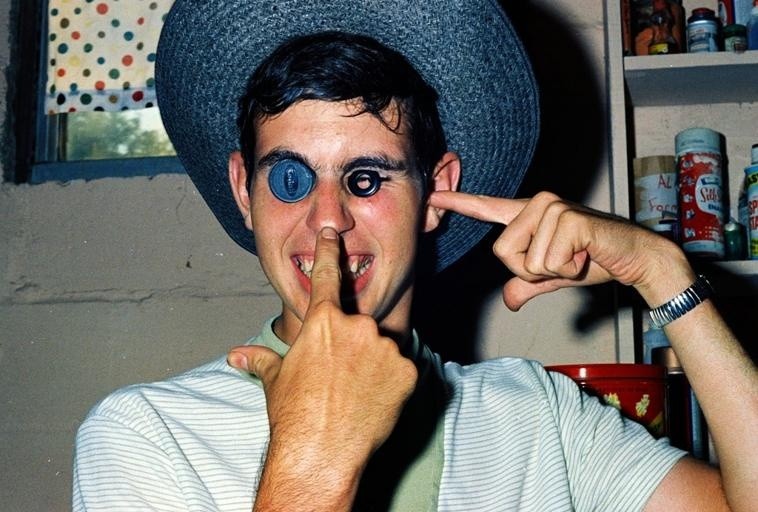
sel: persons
[70,0,758,512]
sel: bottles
[642,320,709,465]
[671,127,758,261]
[646,0,758,55]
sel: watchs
[649,271,717,329]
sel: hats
[153,1,542,285]
